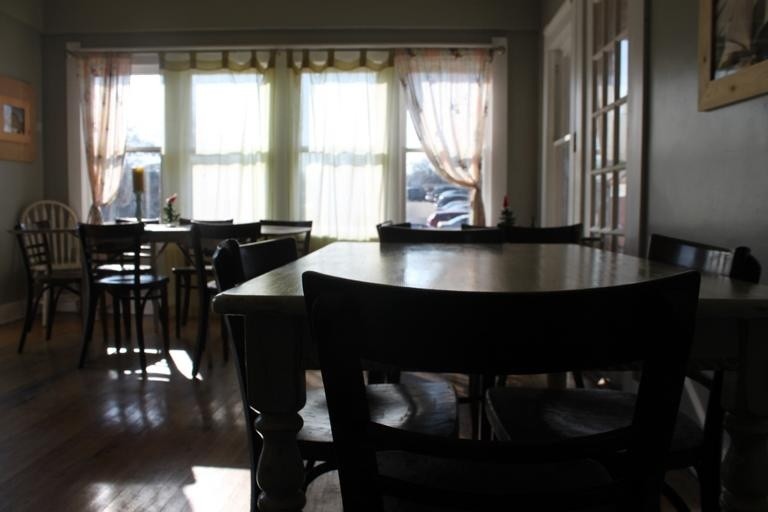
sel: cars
[406,171,470,231]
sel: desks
[212,241,767,512]
[77,222,234,361]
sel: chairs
[15,220,111,356]
[379,221,585,398]
[211,232,460,512]
[258,220,314,259]
[94,217,159,344]
[484,232,761,512]
[171,218,233,340]
[300,268,704,512]
[78,221,170,371]
[191,221,265,379]
[20,199,80,343]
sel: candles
[132,168,145,193]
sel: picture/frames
[0,95,31,144]
[696,0,768,113]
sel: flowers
[498,193,516,227]
[162,193,181,223]
